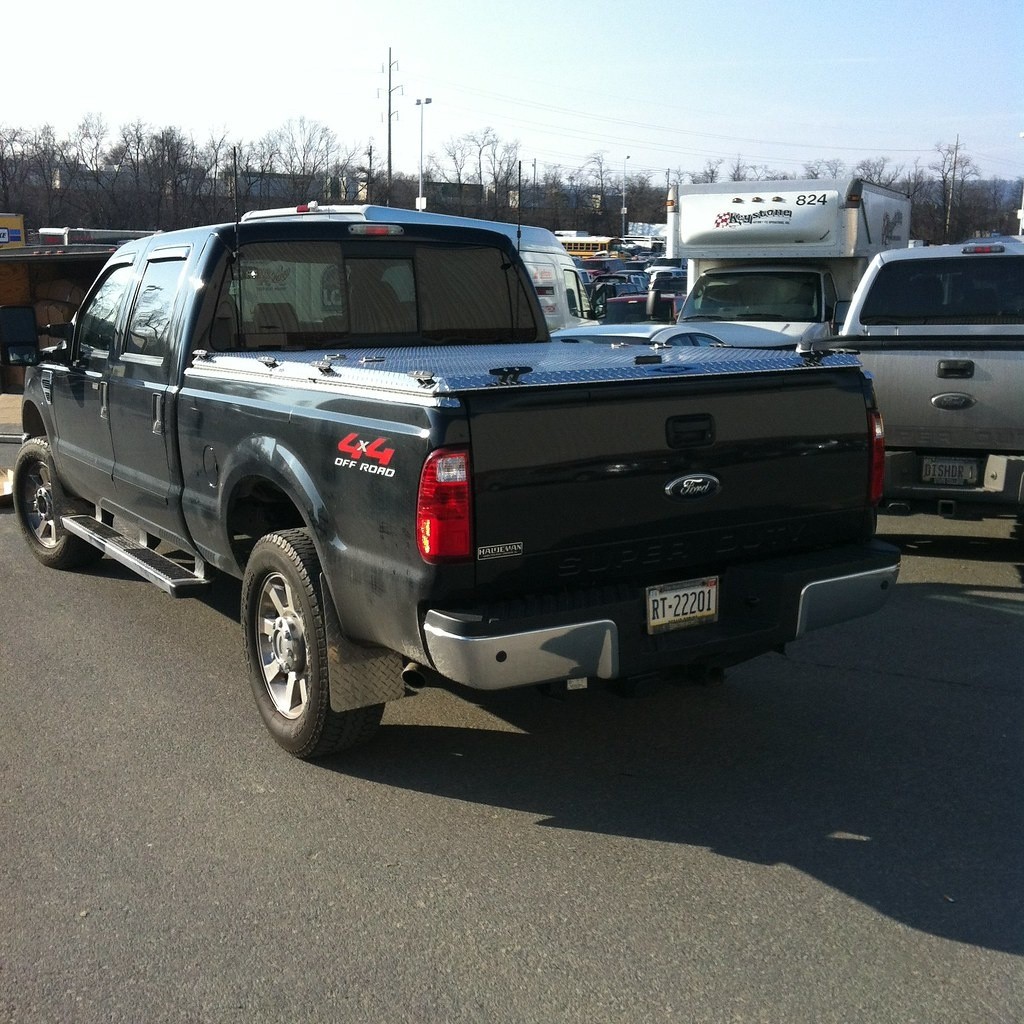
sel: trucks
[664,177,909,356]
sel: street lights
[622,155,630,238]
[415,97,432,212]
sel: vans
[602,290,689,326]
[240,203,600,350]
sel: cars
[580,251,686,277]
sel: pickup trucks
[0,216,900,767]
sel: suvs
[832,241,1023,524]
[592,283,647,304]
[592,274,648,292]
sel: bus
[558,236,625,259]
